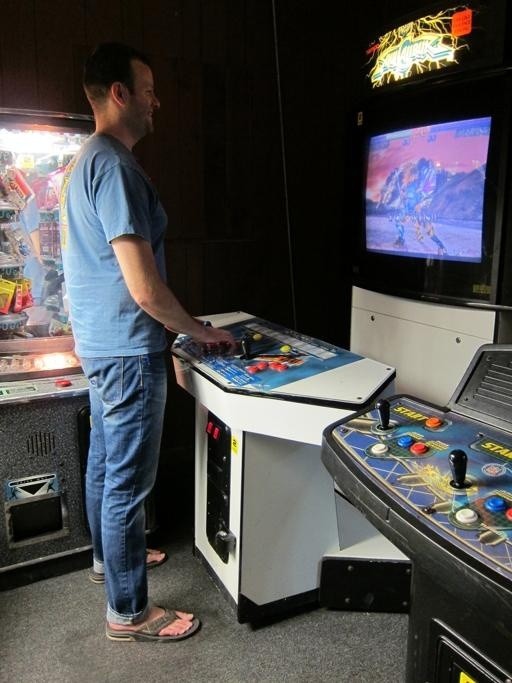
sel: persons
[58,43,235,645]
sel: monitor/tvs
[339,74,506,302]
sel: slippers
[104,605,200,644]
[88,549,167,585]
